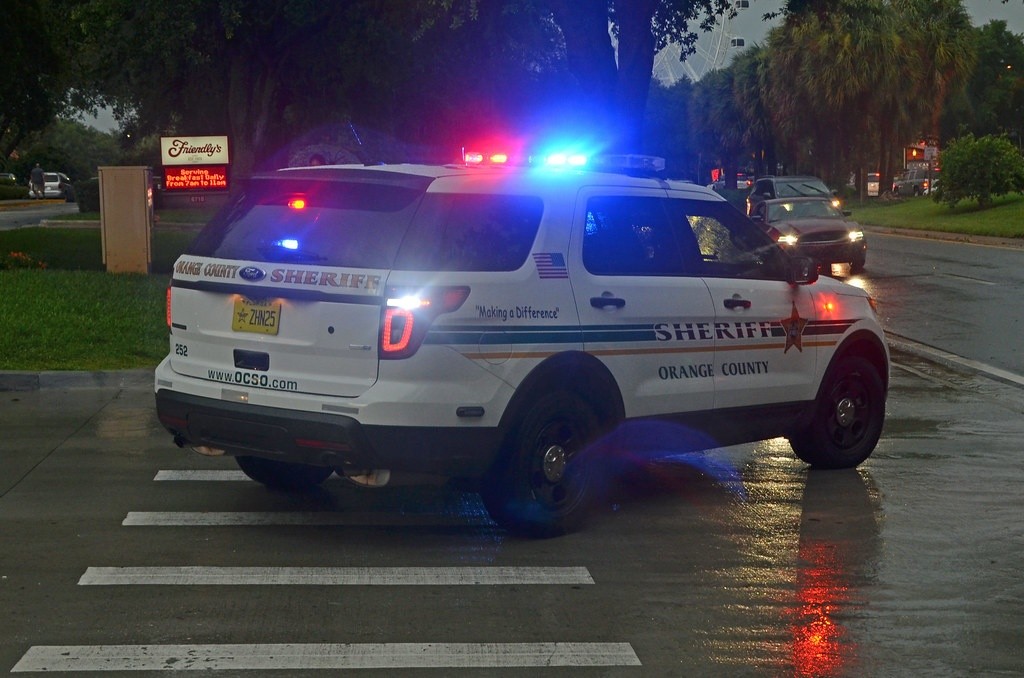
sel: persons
[311,155,325,166]
[850,172,856,183]
[31,163,46,200]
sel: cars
[0,173,18,185]
[753,198,866,270]
[922,182,942,196]
[845,172,880,196]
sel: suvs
[706,171,750,191]
[152,165,892,538]
[29,172,74,202]
[894,167,941,195]
[748,174,843,214]
